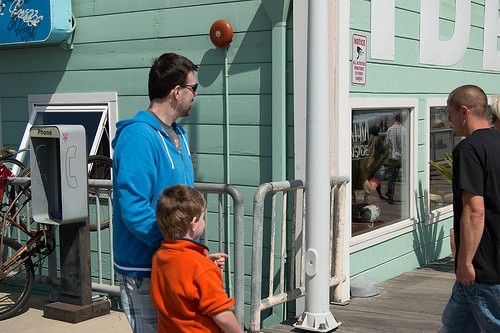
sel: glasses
[180,83,198,92]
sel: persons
[363,111,404,205]
[491,95,500,132]
[150,184,246,333]
[110,53,200,333]
[441,85,500,333]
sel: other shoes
[385,196,394,205]
[379,195,386,200]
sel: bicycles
[0,147,113,321]
